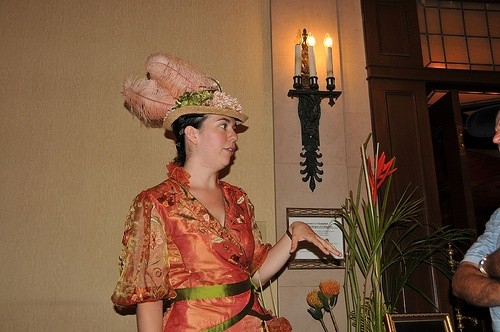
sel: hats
[122,51,249,133]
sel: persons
[453,110,500,332]
[111,53,342,332]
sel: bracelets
[286,229,293,240]
[478,254,490,276]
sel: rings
[325,238,329,243]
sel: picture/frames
[384,313,454,332]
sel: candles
[293,32,335,92]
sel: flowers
[306,134,474,332]
